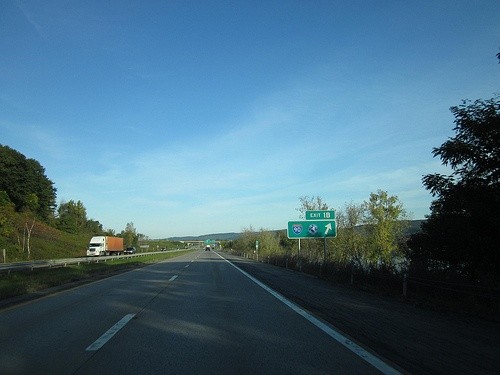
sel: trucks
[206,245,210,251]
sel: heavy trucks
[86,236,124,256]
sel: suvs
[124,247,136,255]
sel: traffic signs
[287,219,337,240]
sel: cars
[161,248,166,251]
